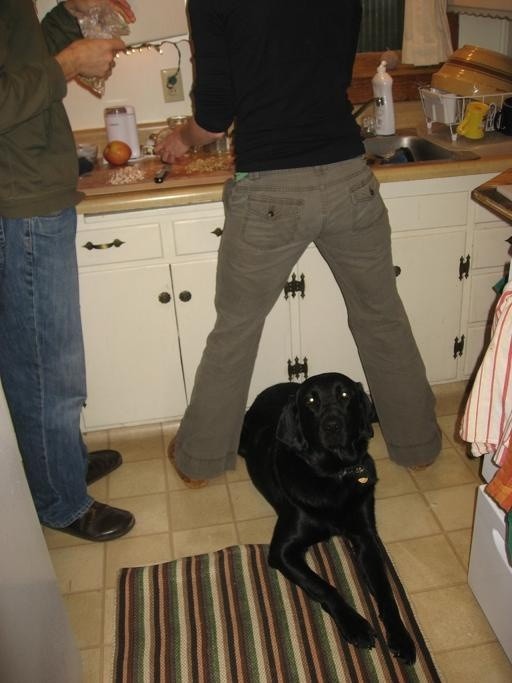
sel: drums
[153,164,173,184]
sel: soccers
[104,141,132,165]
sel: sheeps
[353,96,385,121]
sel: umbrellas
[85,450,122,486]
[168,438,209,488]
[40,500,135,542]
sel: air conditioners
[238,370,417,667]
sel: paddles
[113,526,445,683]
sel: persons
[0,0,135,541]
[169,0,441,489]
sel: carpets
[113,526,445,683]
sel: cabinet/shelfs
[296,176,470,394]
[70,200,297,435]
[459,175,510,379]
[103,106,141,160]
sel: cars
[456,102,490,140]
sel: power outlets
[160,68,184,103]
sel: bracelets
[75,143,98,163]
[430,44,511,109]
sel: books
[466,485,511,664]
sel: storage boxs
[466,485,511,664]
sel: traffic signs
[160,68,184,103]
